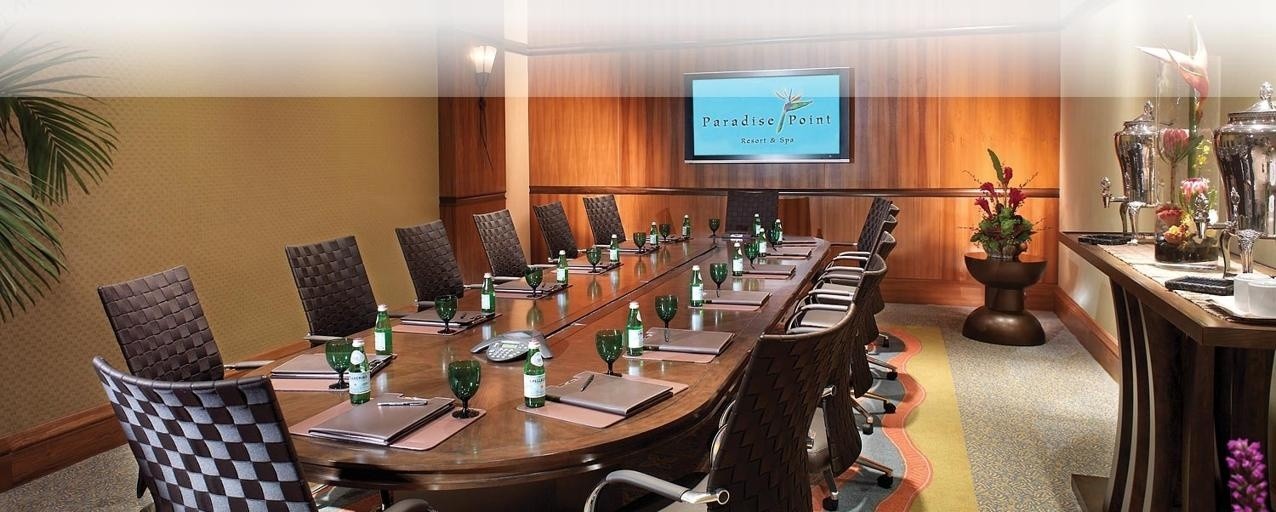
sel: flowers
[1223,435,1273,511]
[953,150,1059,259]
[1140,23,1218,246]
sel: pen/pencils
[580,374,594,392]
[377,401,428,406]
[600,259,603,265]
[542,282,546,288]
[461,313,467,319]
[750,264,755,270]
[665,328,669,342]
[671,235,675,238]
[716,291,720,297]
[773,247,777,252]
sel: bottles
[373,303,395,357]
[773,216,783,247]
[348,337,372,405]
[751,214,762,238]
[680,214,692,240]
[624,300,645,356]
[524,339,548,409]
[609,233,622,267]
[756,226,766,260]
[729,237,744,278]
[686,264,706,310]
[649,221,659,246]
[554,250,569,285]
[480,273,496,317]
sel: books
[613,242,655,252]
[781,236,816,243]
[644,325,738,355]
[701,290,770,305]
[1078,231,1132,246]
[557,260,615,272]
[495,278,567,293]
[743,264,796,275]
[767,247,812,256]
[540,369,674,417]
[1166,274,1234,296]
[400,308,482,329]
[309,396,455,448]
[269,353,394,381]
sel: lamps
[465,40,499,91]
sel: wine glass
[707,215,720,238]
[708,259,728,300]
[596,327,624,379]
[523,266,544,299]
[633,231,647,255]
[654,294,678,343]
[768,227,778,251]
[445,361,481,420]
[742,240,759,270]
[434,294,458,334]
[323,337,354,389]
[658,222,671,243]
[584,247,601,274]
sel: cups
[1248,283,1276,316]
[1233,274,1272,310]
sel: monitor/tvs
[682,66,851,164]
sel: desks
[1060,229,1276,512]
[239,228,832,512]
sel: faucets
[1193,194,1228,238]
[1100,175,1126,209]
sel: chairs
[798,201,899,512]
[96,264,228,383]
[395,220,480,310]
[584,191,626,245]
[96,353,429,512]
[477,210,553,284]
[723,186,779,233]
[533,201,595,265]
[580,306,855,512]
[283,233,402,346]
